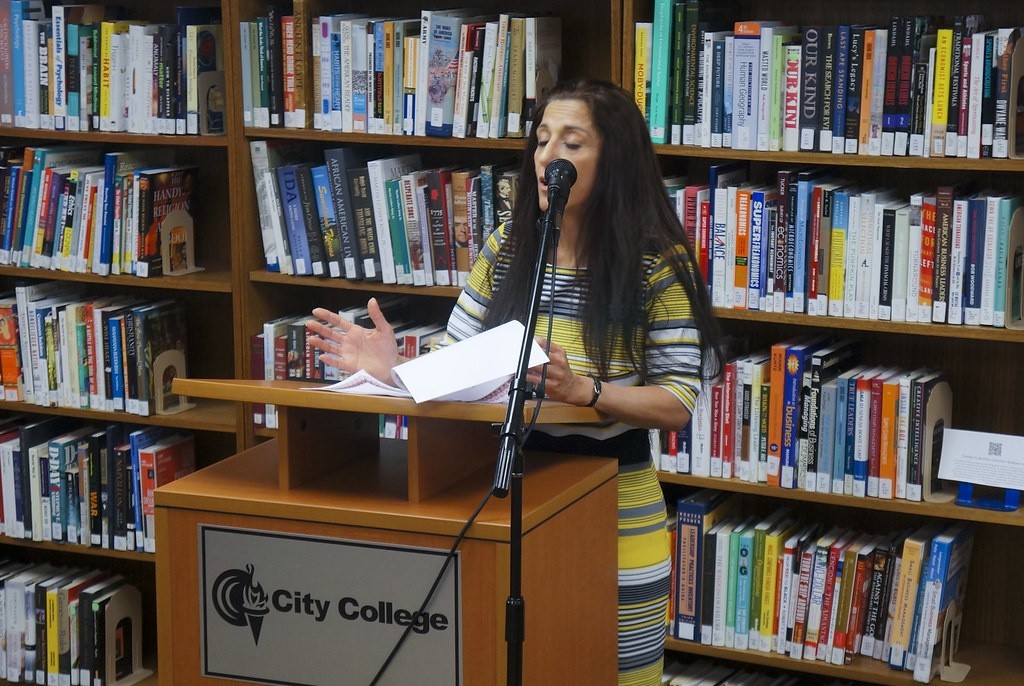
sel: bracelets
[586,374,602,408]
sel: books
[1,0,1024,686]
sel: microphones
[545,158,578,247]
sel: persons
[305,79,725,686]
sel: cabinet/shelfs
[0,1,1024,686]
